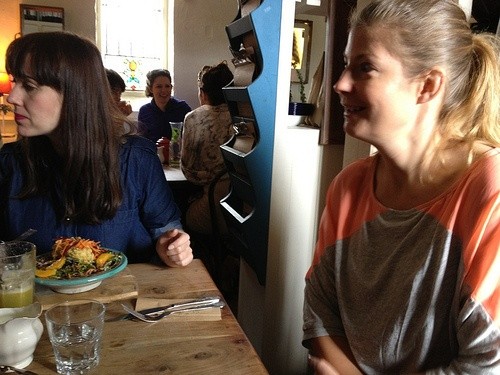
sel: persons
[105,68,133,119]
[181,63,242,274]
[0,32,193,267]
[138,69,192,145]
[301,0,500,375]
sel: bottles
[169,122,183,167]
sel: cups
[156,137,169,164]
[0,242,36,308]
[45,299,106,375]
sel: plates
[34,247,128,293]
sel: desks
[24,258,269,375]
[162,163,193,193]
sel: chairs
[194,170,232,265]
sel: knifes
[121,296,220,320]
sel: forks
[121,301,227,322]
